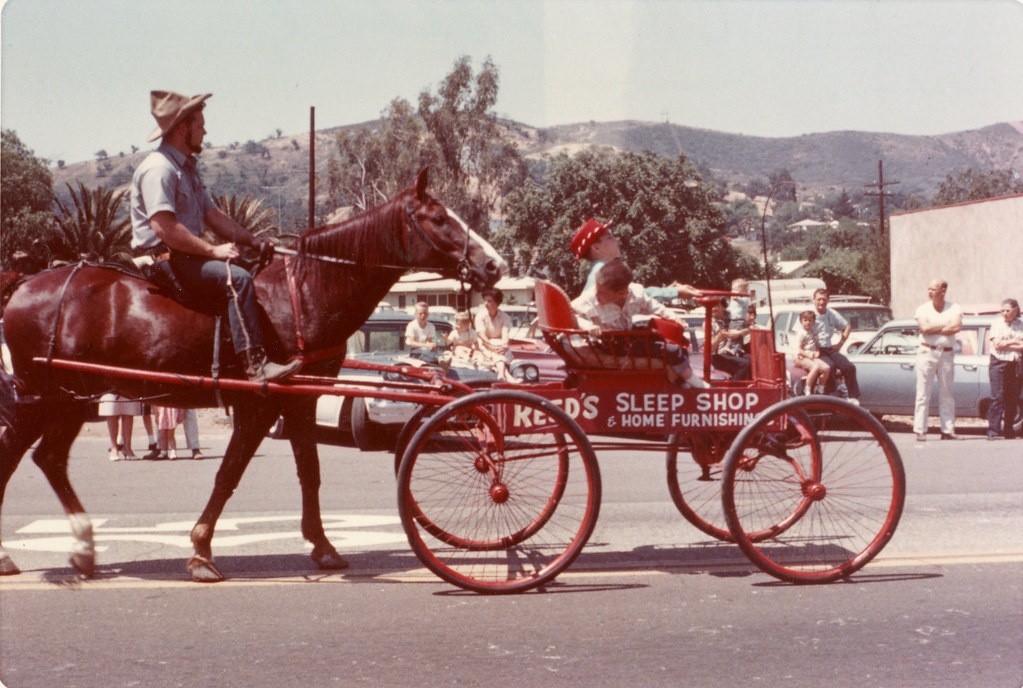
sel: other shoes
[917,433,926,441]
[511,378,523,385]
[247,359,303,384]
[143,450,167,460]
[192,449,204,460]
[1005,434,1018,440]
[988,435,1005,440]
[941,432,963,441]
[846,397,860,407]
[497,373,506,383]
[158,451,167,458]
[168,450,176,460]
[437,356,452,370]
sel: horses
[0,166,505,583]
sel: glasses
[1001,306,1019,313]
[928,287,941,291]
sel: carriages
[0,164,905,595]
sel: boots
[684,373,710,389]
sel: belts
[921,342,953,352]
[132,243,171,258]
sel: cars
[269,308,497,450]
[503,293,1023,438]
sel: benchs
[534,278,677,389]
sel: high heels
[110,448,119,461]
[121,447,138,460]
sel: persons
[810,289,862,407]
[404,288,526,388]
[98,392,215,461]
[791,312,830,396]
[130,90,300,385]
[702,298,759,381]
[567,219,715,389]
[912,278,963,440]
[986,299,1023,440]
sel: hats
[570,215,615,264]
[146,90,213,143]
